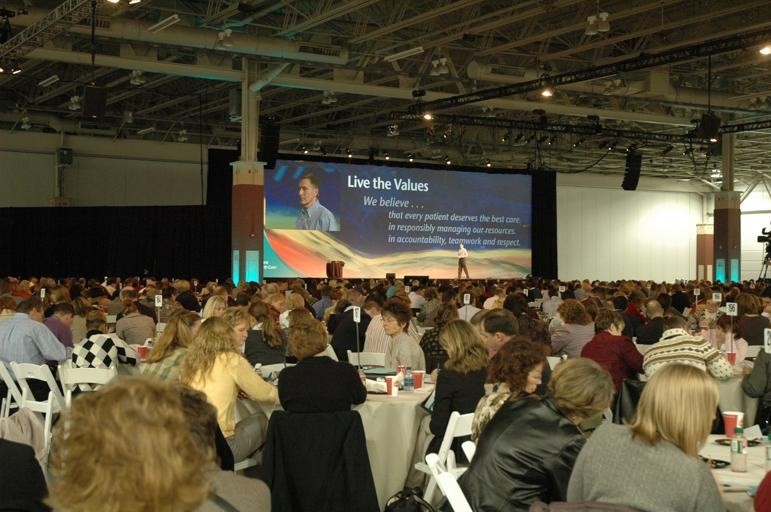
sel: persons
[702,314,748,364]
[222,306,248,349]
[67,310,140,394]
[457,243,470,279]
[157,275,228,316]
[116,276,157,286]
[331,300,380,360]
[480,308,551,394]
[179,317,277,482]
[565,362,724,512]
[228,276,363,308]
[115,286,157,323]
[502,274,578,299]
[0,437,52,512]
[72,275,116,309]
[0,295,72,413]
[741,277,771,293]
[470,336,544,446]
[141,309,202,384]
[43,303,75,351]
[636,300,668,345]
[671,279,689,312]
[419,303,459,373]
[423,318,490,454]
[440,356,615,512]
[642,314,732,385]
[689,280,740,315]
[277,318,366,413]
[363,277,503,310]
[0,275,72,302]
[550,299,595,360]
[295,175,336,231]
[732,293,771,347]
[380,299,426,373]
[580,310,643,417]
[39,374,220,512]
[579,279,636,310]
[636,280,671,305]
[245,301,285,365]
[287,307,340,361]
[503,294,550,343]
[742,346,771,431]
[116,300,157,347]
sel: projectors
[695,112,722,139]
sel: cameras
[757,228,771,256]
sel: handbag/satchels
[383,486,435,512]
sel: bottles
[403,366,413,391]
[730,427,748,472]
[763,434,771,471]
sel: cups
[726,352,737,367]
[721,409,746,438]
[384,375,401,397]
[136,345,150,362]
[394,365,406,378]
[409,367,426,391]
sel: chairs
[271,414,361,511]
[56,363,118,409]
[0,361,21,416]
[13,361,64,450]
[414,408,490,502]
[426,439,477,512]
[231,458,257,475]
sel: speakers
[621,152,642,191]
[260,115,280,169]
[81,86,106,129]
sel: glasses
[380,316,402,323]
